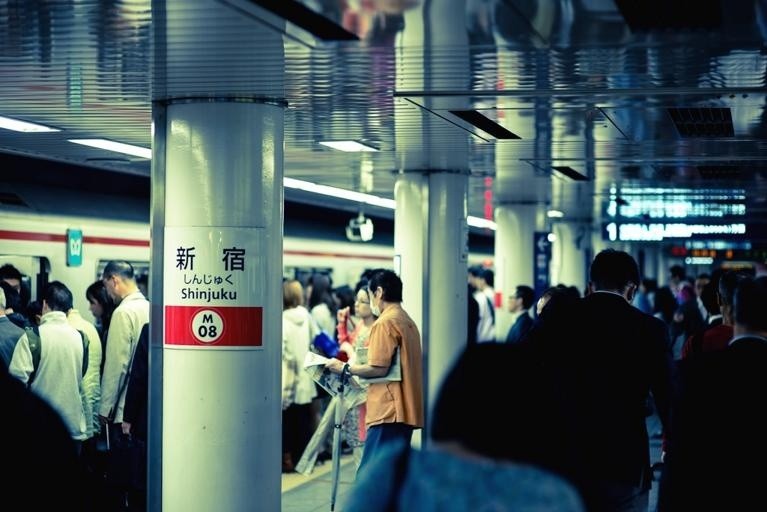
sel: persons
[0,247,149,510]
[631,264,766,510]
[531,249,671,510]
[341,338,584,509]
[506,284,583,341]
[281,269,424,478]
[467,266,498,344]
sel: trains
[1,206,494,335]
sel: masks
[370,290,381,317]
